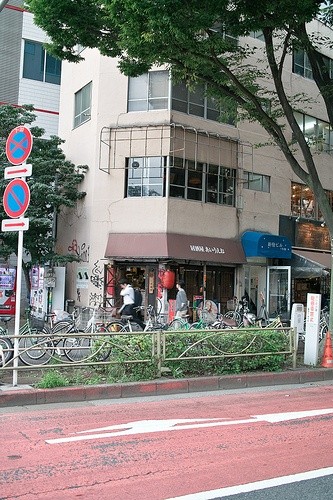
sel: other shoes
[143,325,146,330]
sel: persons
[119,279,146,335]
[175,280,187,329]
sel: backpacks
[127,286,142,306]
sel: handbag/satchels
[187,306,192,317]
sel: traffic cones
[321,331,333,368]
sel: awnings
[242,231,291,258]
[104,233,247,264]
[292,249,331,278]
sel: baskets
[227,300,236,311]
[281,312,290,322]
[29,311,46,329]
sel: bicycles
[0,296,329,369]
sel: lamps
[190,173,201,185]
[132,162,139,169]
[321,222,326,227]
[296,216,300,223]
